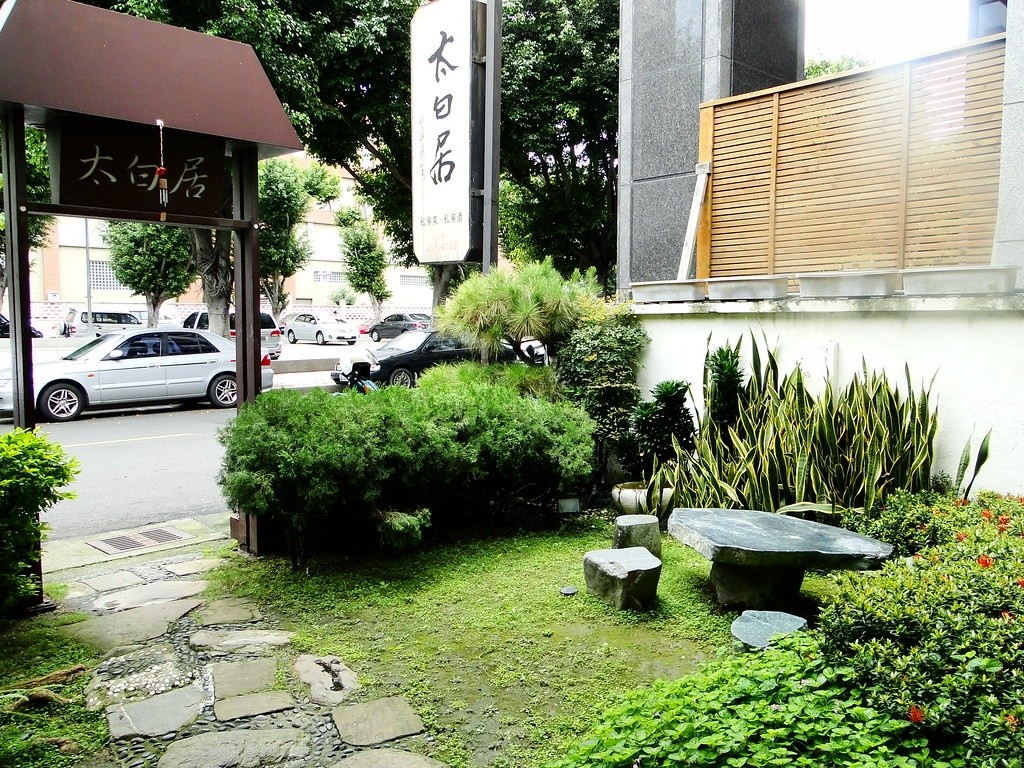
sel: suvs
[59,308,150,338]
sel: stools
[583,547,661,611]
[730,610,808,651]
[610,514,662,560]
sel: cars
[278,313,299,336]
[283,312,361,344]
[0,327,274,423]
[498,334,545,364]
[329,327,520,387]
[0,312,44,338]
[369,313,433,342]
[360,316,384,335]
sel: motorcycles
[336,345,379,397]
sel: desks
[668,508,893,611]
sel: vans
[181,309,283,361]
